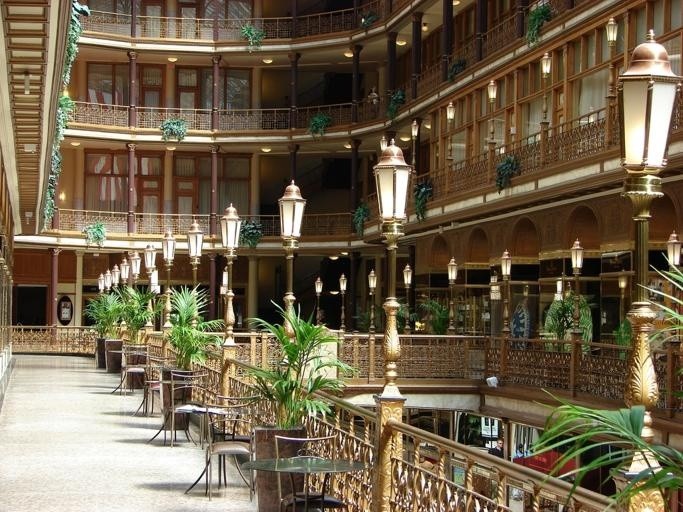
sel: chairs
[274,435,336,512]
[148,369,214,450]
[111,344,177,415]
[185,395,261,503]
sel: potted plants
[230,300,354,511]
[123,289,165,389]
[86,284,134,373]
[162,283,227,430]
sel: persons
[516,445,530,458]
[493,438,504,457]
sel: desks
[239,458,372,512]
